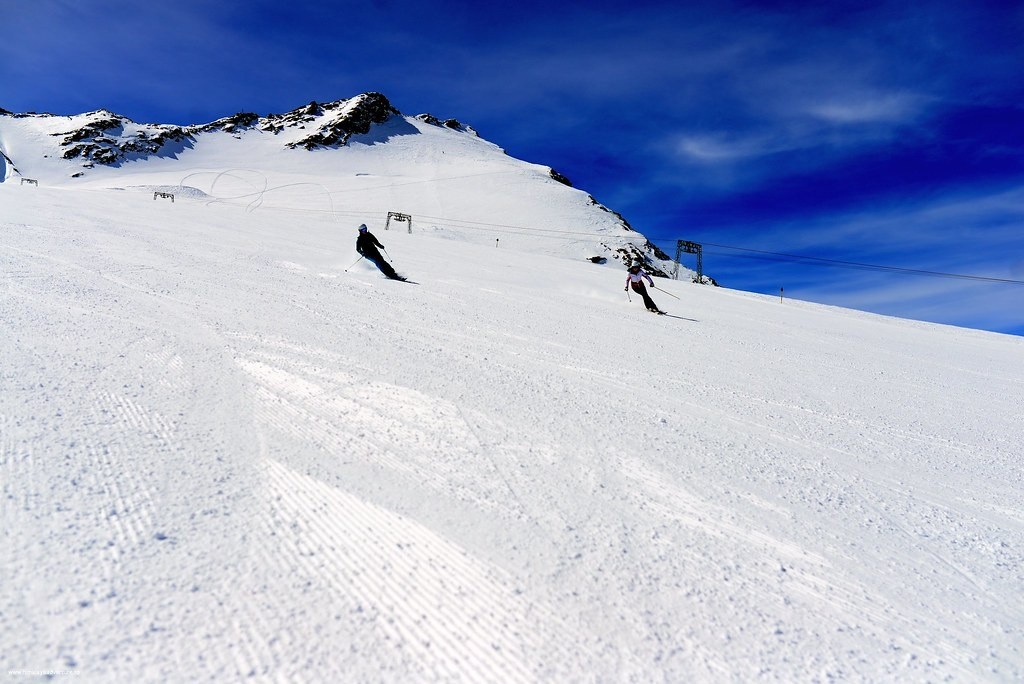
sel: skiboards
[650,309,667,315]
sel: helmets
[358,224,367,231]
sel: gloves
[625,288,628,291]
[650,283,654,287]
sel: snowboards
[386,277,418,284]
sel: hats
[630,262,640,267]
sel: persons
[624,261,666,315]
[356,224,407,282]
